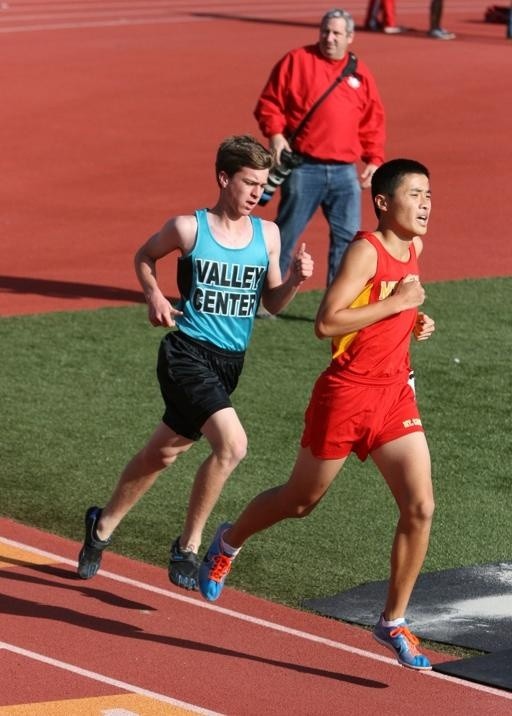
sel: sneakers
[166,534,200,591]
[370,613,435,672]
[198,523,243,603]
[429,27,455,39]
[76,505,109,579]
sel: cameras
[257,150,304,207]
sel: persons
[251,6,389,319]
[428,0,457,41]
[75,133,317,590]
[363,0,406,35]
[198,157,437,672]
